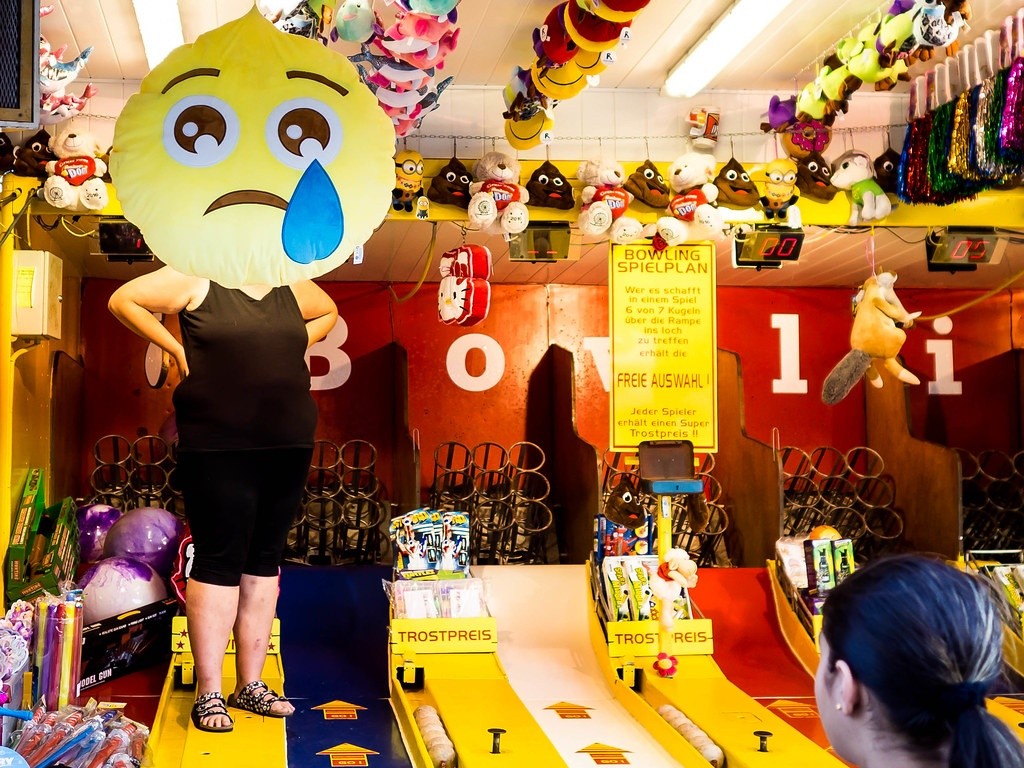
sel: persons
[107,265,338,733]
[814,555,1024,768]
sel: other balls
[73,504,186,625]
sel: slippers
[226,681,293,718]
[191,691,235,732]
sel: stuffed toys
[259,0,462,138]
[760,0,973,159]
[648,548,698,629]
[0,5,112,211]
[503,0,650,150]
[393,149,901,251]
[437,244,492,327]
[605,474,645,530]
[822,265,922,406]
[653,653,678,678]
[687,493,710,535]
[108,0,396,288]
